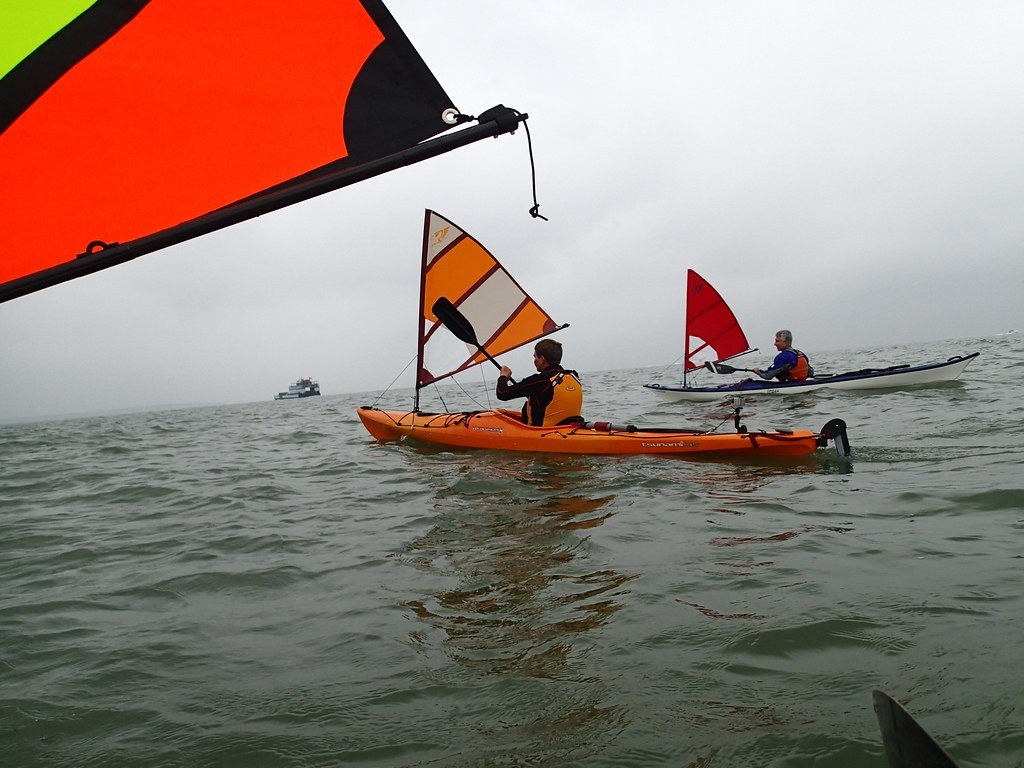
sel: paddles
[433,296,518,386]
[705,360,763,374]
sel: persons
[754,330,814,382]
[496,339,584,427]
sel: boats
[274,377,320,400]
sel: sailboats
[644,270,981,404]
[358,209,830,459]
